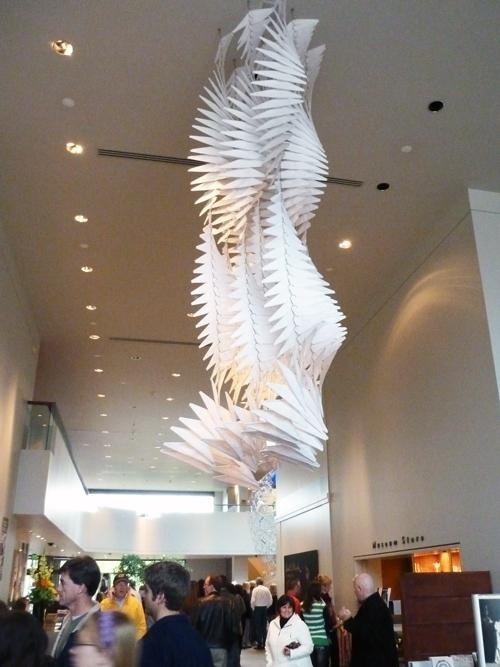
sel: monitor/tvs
[471,592,500,667]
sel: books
[408,652,474,666]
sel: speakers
[429,656,454,667]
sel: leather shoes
[242,641,265,650]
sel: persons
[180,572,402,665]
[96,572,155,641]
[14,596,30,612]
[51,554,102,666]
[64,608,136,667]
[0,607,62,666]
[127,559,216,666]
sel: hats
[114,573,129,584]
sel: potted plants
[27,586,57,627]
